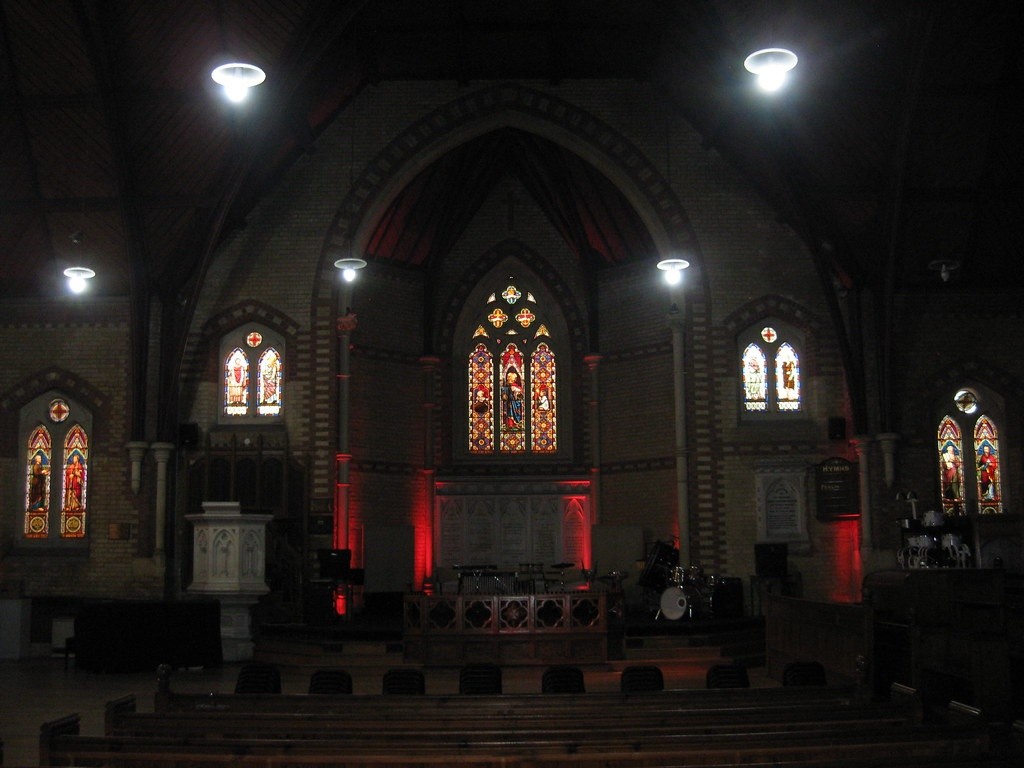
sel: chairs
[542,664,584,694]
[706,663,751,689]
[459,663,502,696]
[383,669,425,696]
[783,661,828,687]
[234,662,281,694]
[309,668,352,694]
[621,664,664,692]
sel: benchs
[39,654,1024,768]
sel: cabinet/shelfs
[901,519,976,567]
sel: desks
[64,595,222,674]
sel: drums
[660,561,729,621]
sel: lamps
[210,59,266,104]
[744,47,798,94]
[62,234,95,299]
[653,103,694,287]
[334,71,368,285]
